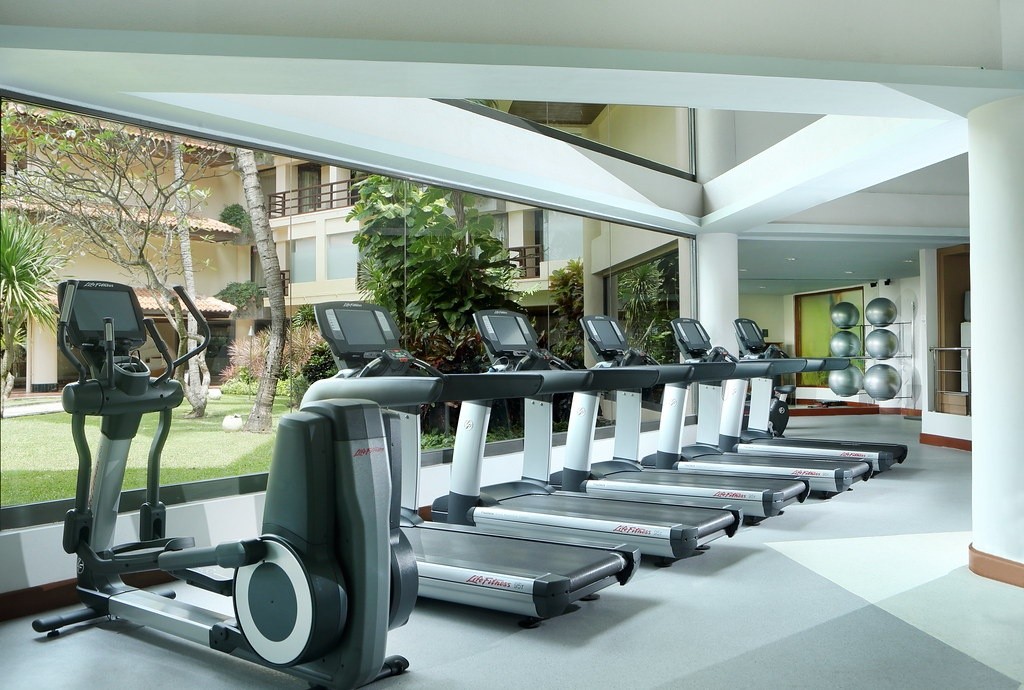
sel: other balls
[827,296,903,402]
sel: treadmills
[55,276,909,690]
